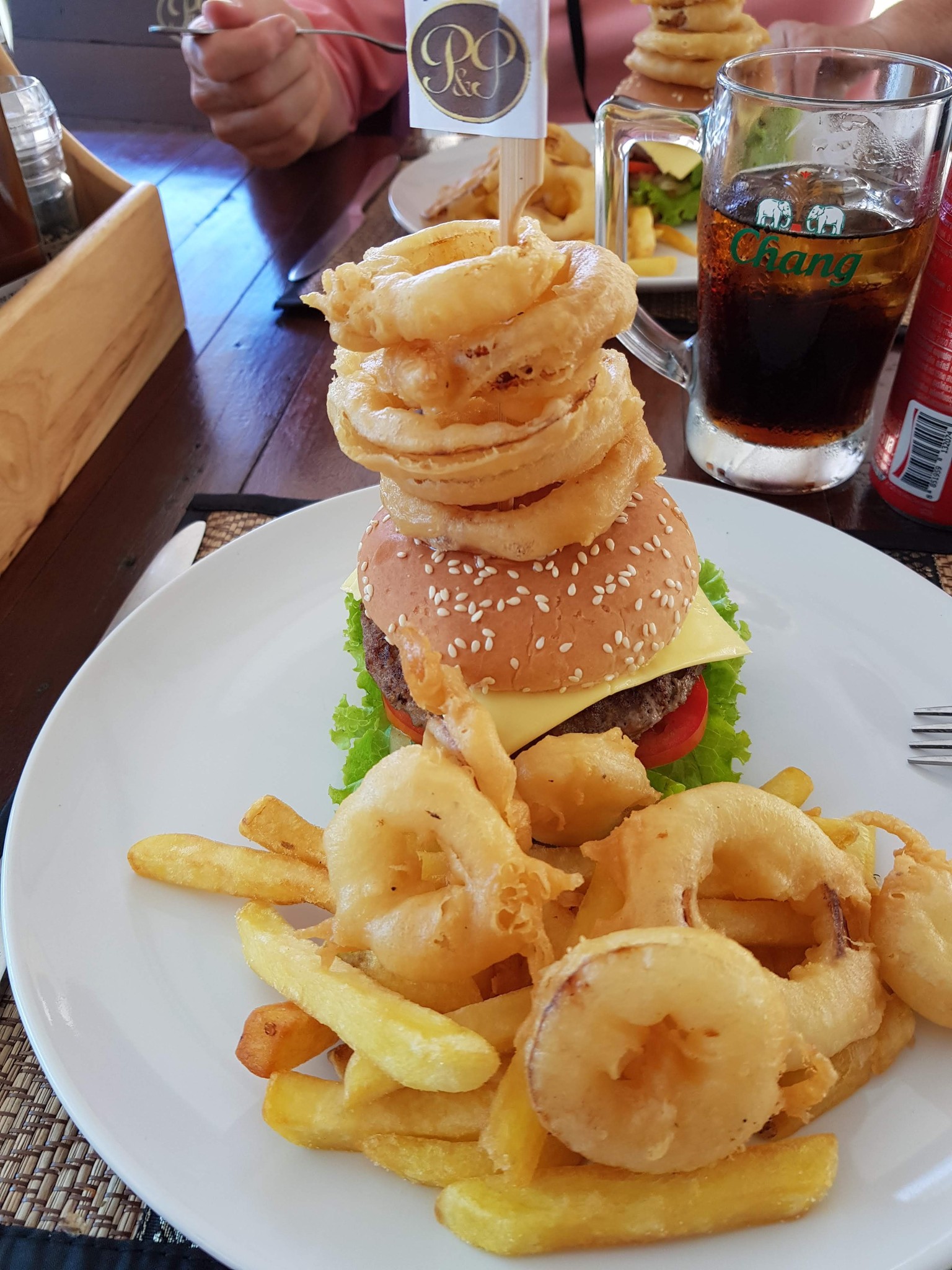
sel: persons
[180,0,951,170]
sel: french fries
[123,766,917,1258]
[627,197,698,277]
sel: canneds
[868,158,952,532]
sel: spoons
[146,26,405,55]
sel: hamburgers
[329,476,752,809]
[612,73,802,227]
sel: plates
[385,123,704,289]
[6,473,952,1270]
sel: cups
[590,45,950,494]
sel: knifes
[98,520,208,656]
[287,153,400,281]
